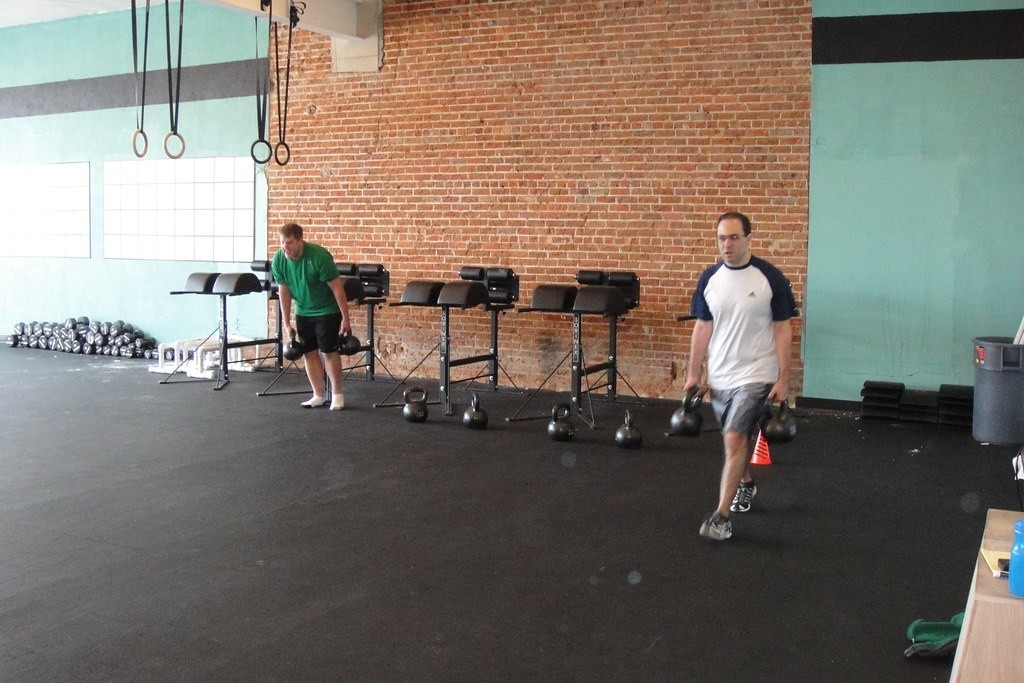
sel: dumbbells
[6,317,195,360]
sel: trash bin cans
[970,335,1024,447]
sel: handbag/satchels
[904,612,965,658]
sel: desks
[949,508,1024,682]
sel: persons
[682,212,799,540]
[271,224,351,410]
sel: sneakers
[699,512,732,540]
[730,477,757,512]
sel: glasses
[716,234,745,243]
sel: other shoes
[300,396,325,408]
[329,394,344,410]
